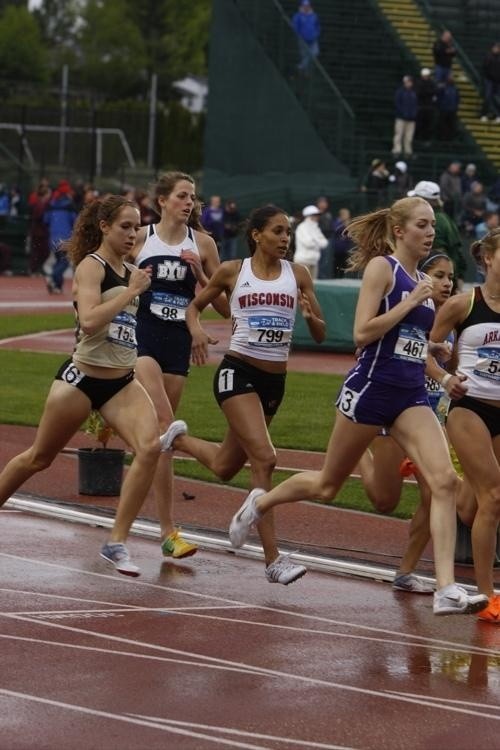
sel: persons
[112,185,159,220]
[481,45,499,122]
[81,269,83,273]
[433,30,457,79]
[360,251,460,594]
[198,196,238,254]
[2,193,161,575]
[293,2,319,73]
[124,173,228,558]
[55,180,105,210]
[226,197,489,616]
[360,160,495,230]
[2,180,48,233]
[437,75,459,136]
[419,70,437,140]
[150,203,327,584]
[390,76,420,154]
[43,190,77,295]
[293,190,356,277]
[426,233,500,623]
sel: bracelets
[440,374,451,389]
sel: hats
[395,160,411,172]
[59,184,71,192]
[302,203,321,216]
[59,179,65,184]
[407,182,444,203]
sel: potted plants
[449,440,500,566]
[75,410,125,497]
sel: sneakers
[44,275,57,294]
[161,530,201,559]
[265,554,310,586]
[479,597,500,623]
[160,420,189,449]
[99,540,142,577]
[230,486,266,548]
[433,585,487,616]
[391,569,437,597]
